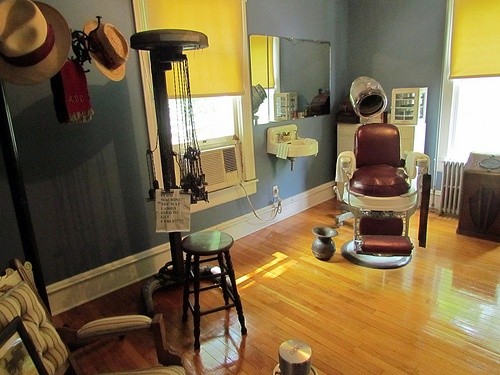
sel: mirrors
[249,35,332,125]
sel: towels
[302,138,318,157]
[405,152,430,179]
[275,141,291,160]
[334,150,356,183]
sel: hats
[0,0,71,85]
[82,20,129,81]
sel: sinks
[275,137,319,158]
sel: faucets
[282,132,290,139]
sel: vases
[311,226,337,263]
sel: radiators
[438,161,463,216]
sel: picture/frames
[0,315,48,375]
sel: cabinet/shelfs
[338,125,427,203]
[391,87,429,125]
[274,90,298,121]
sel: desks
[457,151,500,242]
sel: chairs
[334,124,433,269]
[0,258,196,375]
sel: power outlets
[272,184,281,198]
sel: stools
[179,229,248,354]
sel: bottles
[311,226,339,262]
